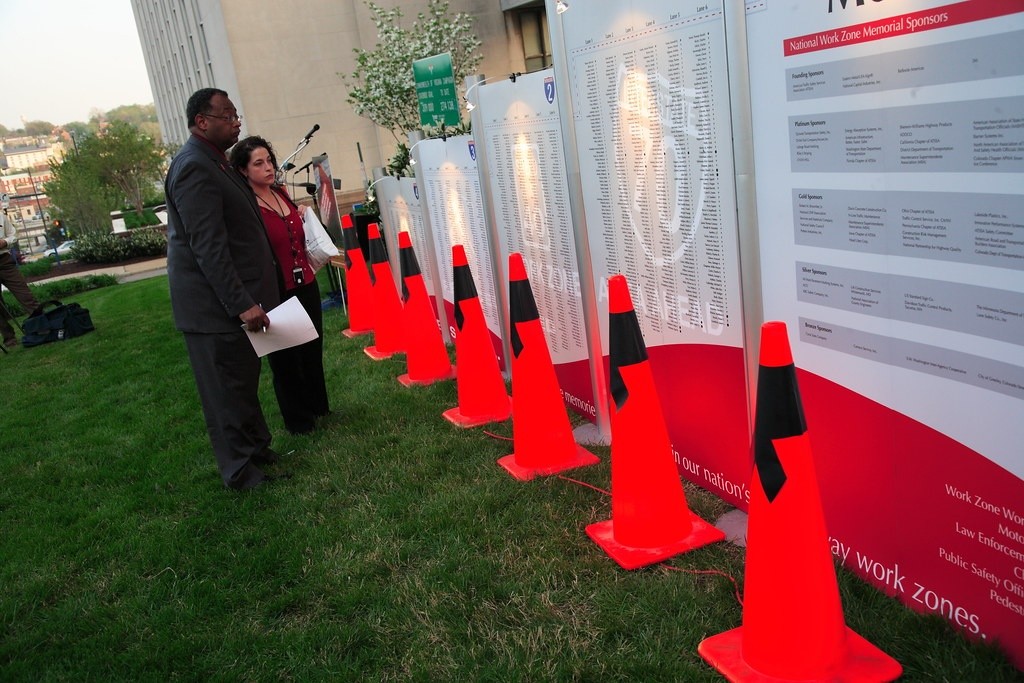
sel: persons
[0,211,40,347]
[230,136,329,434]
[164,88,288,491]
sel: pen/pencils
[259,302,267,333]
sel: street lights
[1,166,61,267]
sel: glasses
[202,114,243,123]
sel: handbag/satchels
[300,207,340,276]
[21,299,95,349]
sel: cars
[43,240,94,260]
[32,212,50,221]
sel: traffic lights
[55,221,61,228]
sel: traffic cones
[396,229,456,388]
[698,320,903,683]
[363,221,405,360]
[337,214,375,339]
[441,243,513,428]
[585,271,728,570]
[496,251,602,483]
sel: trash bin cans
[11,246,22,265]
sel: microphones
[294,152,327,174]
[300,125,320,144]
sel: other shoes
[5,338,17,347]
[238,448,312,499]
